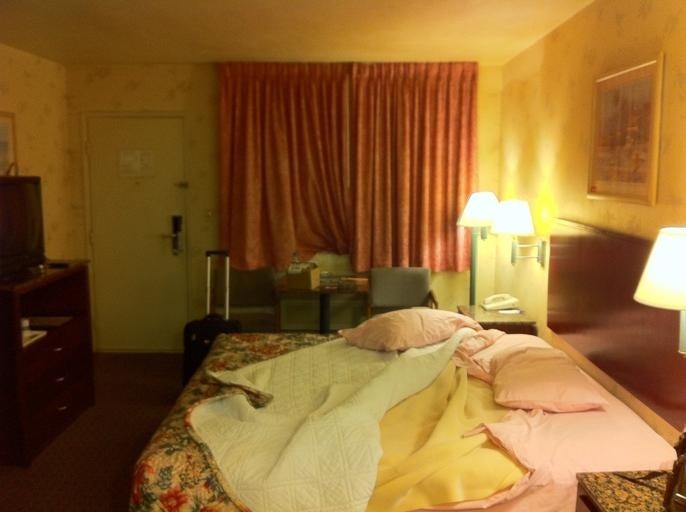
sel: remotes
[49,261,70,268]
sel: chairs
[211,264,280,335]
[359,263,439,323]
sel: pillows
[332,306,486,354]
[488,343,611,414]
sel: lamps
[454,189,548,270]
[630,223,686,363]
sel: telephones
[480,294,518,311]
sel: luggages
[181,249,241,388]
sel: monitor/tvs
[0,176,43,281]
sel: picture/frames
[0,111,21,176]
[582,49,669,210]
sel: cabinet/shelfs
[0,256,100,470]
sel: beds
[122,212,686,510]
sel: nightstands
[455,301,538,338]
[572,467,686,512]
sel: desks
[274,276,370,335]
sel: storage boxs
[283,260,320,292]
